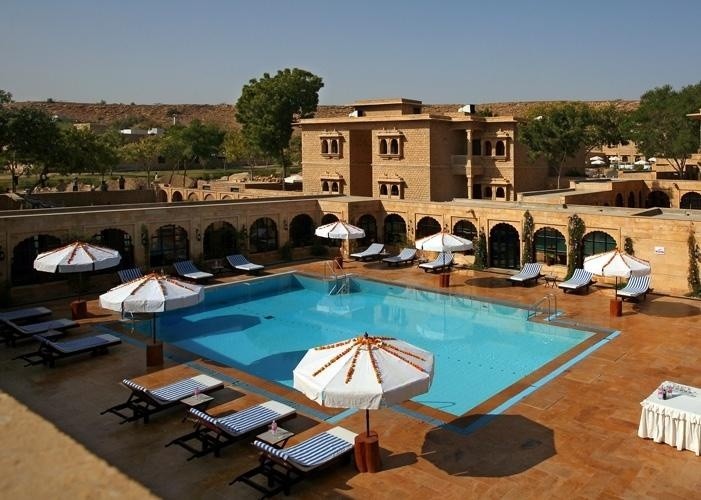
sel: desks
[636,380,701,457]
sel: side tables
[211,266,224,277]
[180,393,216,427]
[256,426,295,449]
[544,275,558,289]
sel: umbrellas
[32,239,123,302]
[583,247,651,300]
[283,174,302,184]
[313,218,366,257]
[292,332,435,437]
[98,269,205,345]
[414,226,474,273]
[590,155,656,165]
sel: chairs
[100,373,224,425]
[225,254,264,275]
[510,262,541,286]
[418,253,454,273]
[230,426,361,500]
[382,248,417,267]
[116,268,143,285]
[0,306,121,367]
[163,399,296,461]
[173,260,214,284]
[350,243,385,262]
[617,275,651,303]
[558,269,593,293]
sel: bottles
[657,383,698,400]
[193,386,200,401]
[271,420,277,436]
